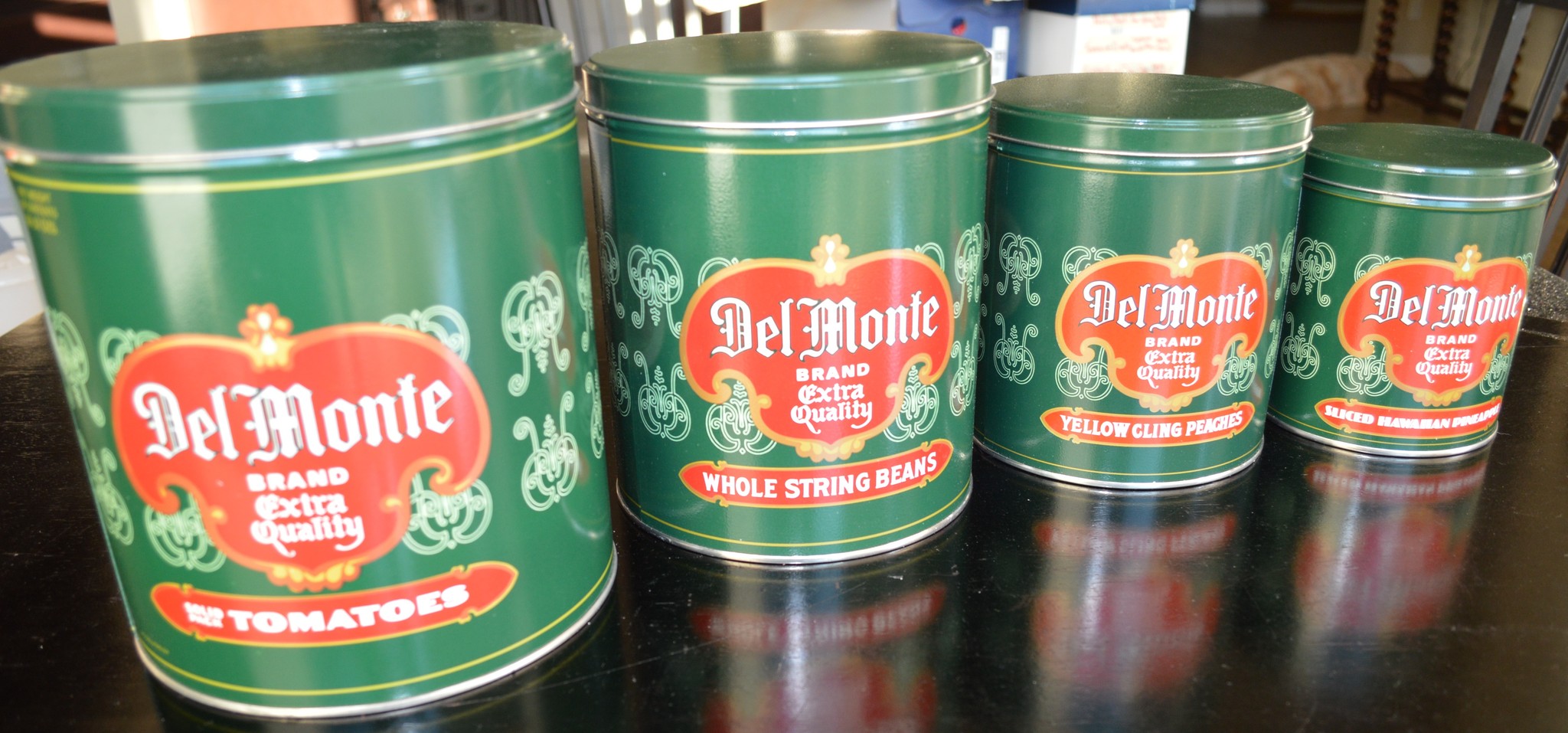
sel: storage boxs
[1017,1,1190,78]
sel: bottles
[976,72,1320,500]
[1267,121,1562,465]
[578,30,994,574]
[0,19,625,724]
